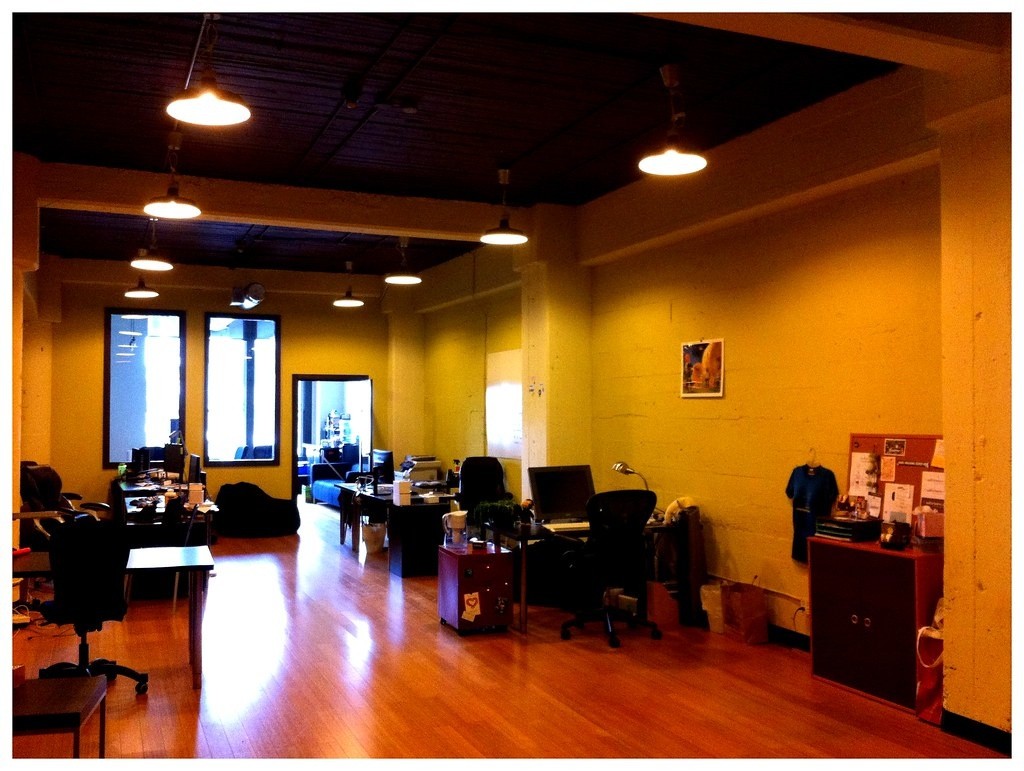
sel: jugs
[443,510,469,549]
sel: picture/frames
[680,338,725,398]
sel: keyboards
[542,522,590,532]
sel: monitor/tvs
[188,454,200,489]
[370,449,393,496]
[527,465,596,521]
[164,443,184,473]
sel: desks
[482,514,672,634]
[387,502,451,578]
[13,544,214,690]
[13,676,106,758]
[334,482,454,570]
[114,477,219,610]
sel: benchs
[309,461,370,508]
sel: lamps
[166,12,253,125]
[385,235,422,286]
[333,286,364,307]
[123,273,160,299]
[131,222,173,271]
[169,429,189,467]
[143,129,201,219]
[612,461,660,525]
[480,169,528,245]
[118,315,147,363]
[637,63,707,175]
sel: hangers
[807,448,821,467]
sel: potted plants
[473,499,520,529]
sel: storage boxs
[917,512,945,538]
[392,480,412,506]
[187,483,204,506]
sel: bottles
[164,488,177,505]
[118,462,127,476]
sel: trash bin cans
[701,585,723,634]
[363,523,386,552]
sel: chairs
[551,489,664,647]
[38,518,148,693]
[20,460,111,551]
[453,457,513,541]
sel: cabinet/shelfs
[437,540,513,635]
[807,535,943,727]
[320,413,352,449]
